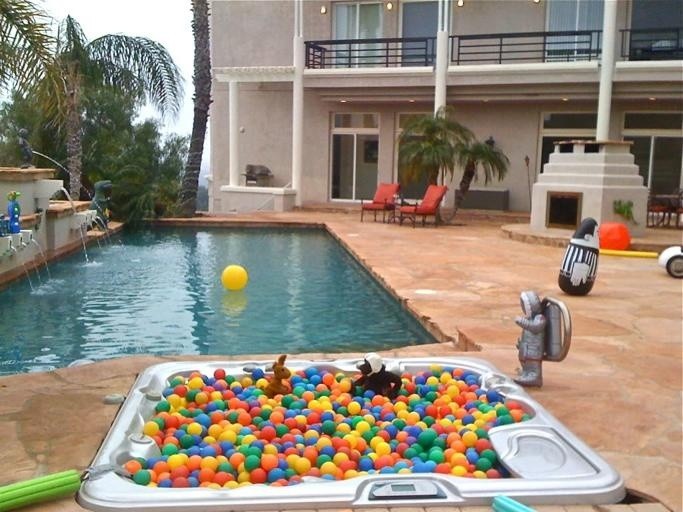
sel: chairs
[400,185,448,227]
[360,183,400,223]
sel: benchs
[629,32,683,60]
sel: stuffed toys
[264,355,291,398]
[353,353,402,402]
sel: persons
[17,128,34,168]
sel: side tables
[387,202,414,228]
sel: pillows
[652,41,675,51]
